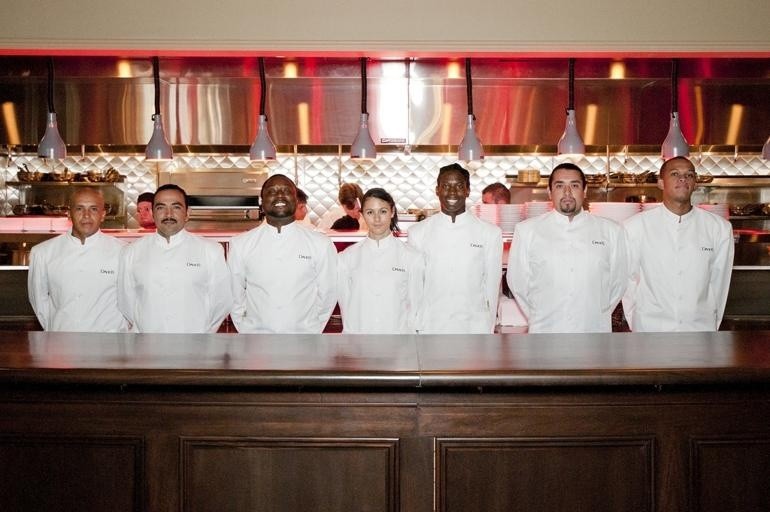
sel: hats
[137,192,155,203]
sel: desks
[0,327,768,510]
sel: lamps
[555,57,588,153]
[661,59,691,162]
[249,58,278,156]
[457,60,483,161]
[348,58,378,156]
[145,57,178,154]
[37,56,71,158]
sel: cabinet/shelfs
[8,182,127,234]
[503,173,770,271]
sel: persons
[227,174,341,334]
[294,188,308,222]
[136,192,155,229]
[116,184,233,333]
[506,163,627,333]
[621,156,734,331]
[408,164,502,334]
[338,188,425,334]
[482,182,510,204]
[28,188,132,333]
[330,183,364,228]
